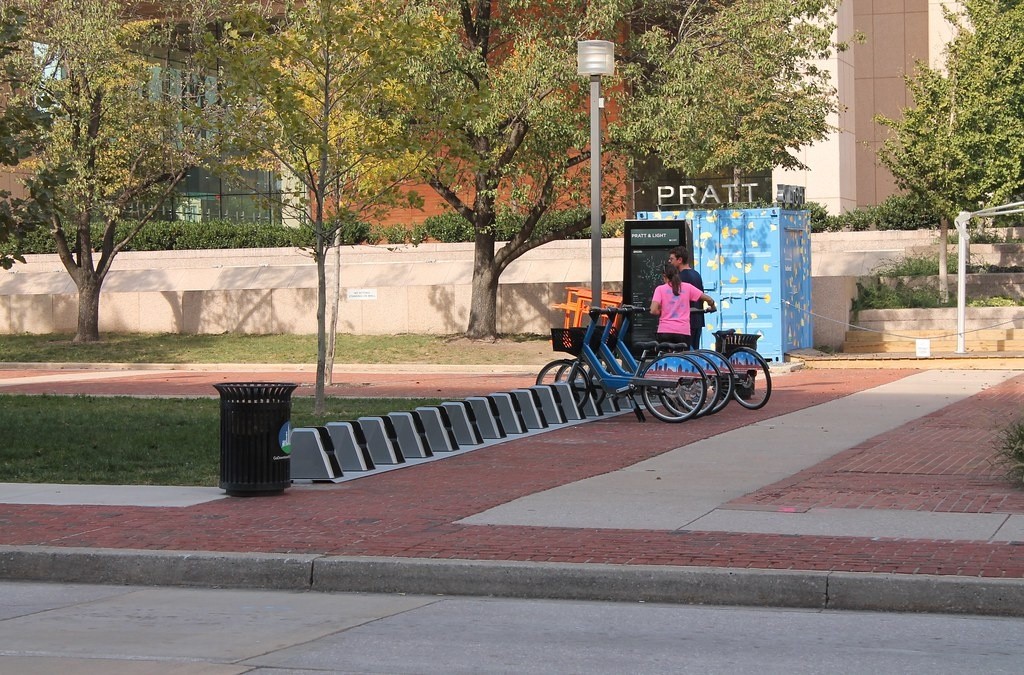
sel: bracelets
[708,301,714,306]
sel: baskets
[584,324,605,352]
[550,327,585,355]
[607,326,619,352]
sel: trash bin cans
[211,381,299,497]
[712,332,761,400]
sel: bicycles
[615,303,733,415]
[690,307,772,409]
[602,306,721,419]
[535,306,704,423]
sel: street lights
[578,40,614,327]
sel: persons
[669,246,705,350]
[650,262,716,352]
